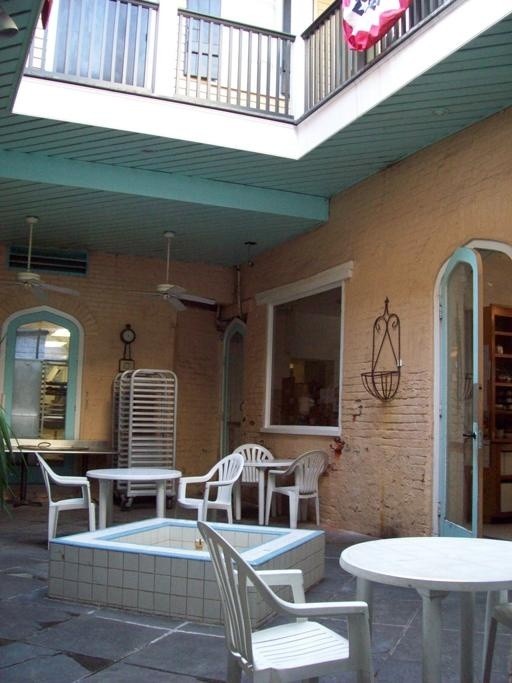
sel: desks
[2,436,119,507]
[84,466,180,528]
[242,459,296,526]
[340,536,512,682]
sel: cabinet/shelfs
[465,442,512,526]
[463,300,512,441]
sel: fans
[124,231,216,312]
[0,216,81,297]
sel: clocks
[121,324,136,344]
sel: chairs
[265,450,329,528]
[173,453,245,523]
[35,452,97,551]
[480,590,511,682]
[196,520,374,683]
[232,442,278,520]
